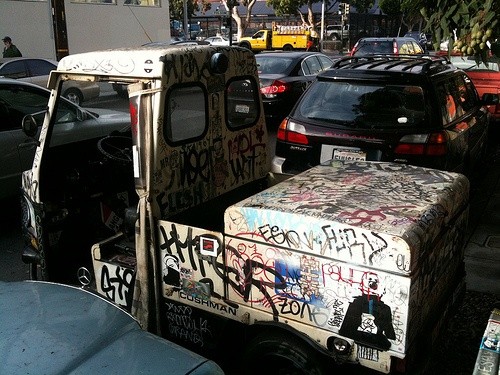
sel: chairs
[270,62,286,75]
[319,91,362,115]
[377,91,412,123]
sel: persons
[2,37,23,57]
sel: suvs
[350,37,425,59]
[270,54,499,181]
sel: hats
[1,36,11,41]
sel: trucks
[18,42,469,375]
[238,21,317,52]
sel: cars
[226,53,337,124]
[0,280,224,375]
[0,79,134,207]
[0,56,101,106]
[403,32,438,51]
[172,20,230,45]
